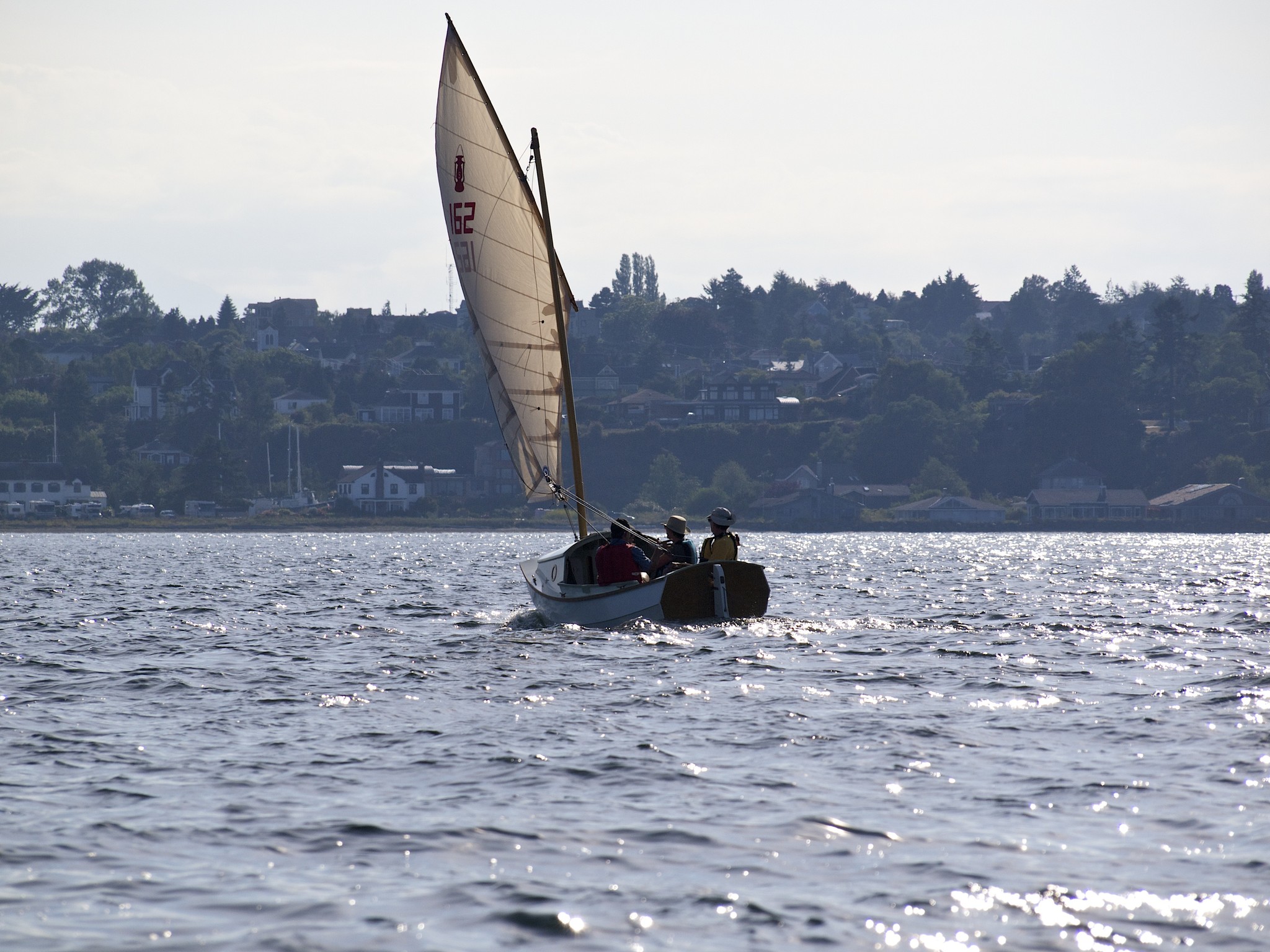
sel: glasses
[707,518,714,524]
[664,525,672,532]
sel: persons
[595,517,672,584]
[628,524,636,544]
[649,515,697,574]
[696,506,739,562]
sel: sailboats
[247,419,337,516]
[433,13,770,626]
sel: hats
[660,515,691,534]
[706,507,737,526]
[611,518,635,533]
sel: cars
[159,510,177,520]
